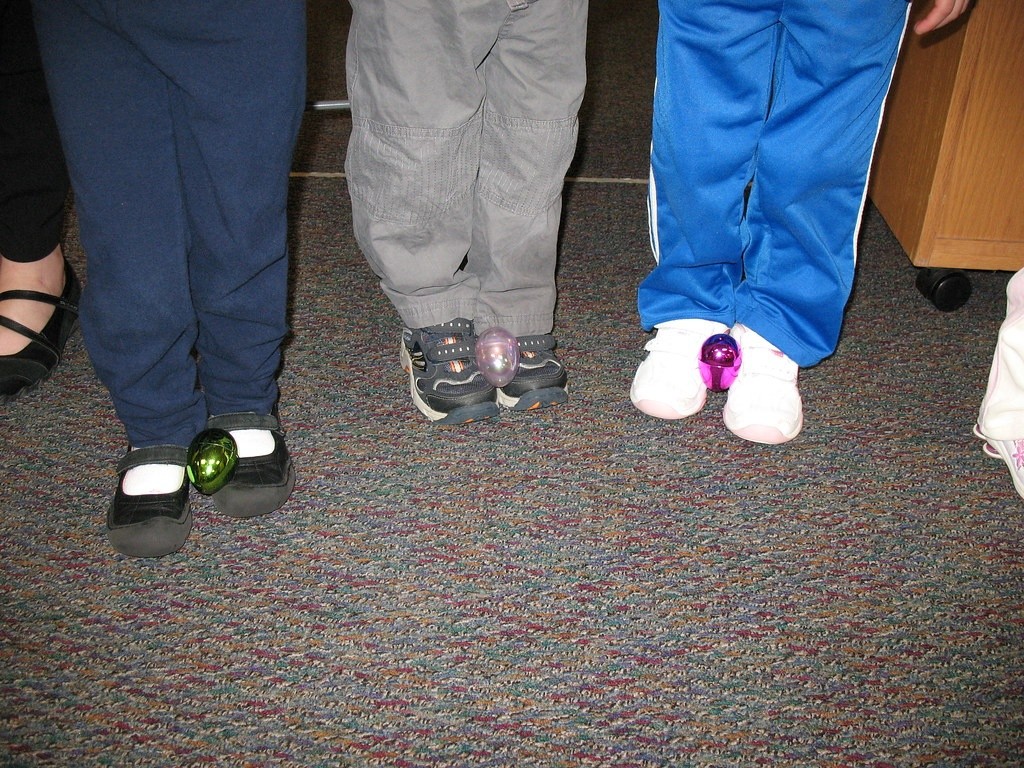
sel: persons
[629,0,969,444]
[32,0,308,558]
[344,0,589,426]
[0,0,82,404]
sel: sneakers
[496,333,569,412]
[630,318,731,419]
[722,320,804,445]
[400,318,500,426]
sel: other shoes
[204,402,297,518]
[105,444,192,558]
[0,255,81,395]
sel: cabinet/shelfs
[867,0,1024,314]
